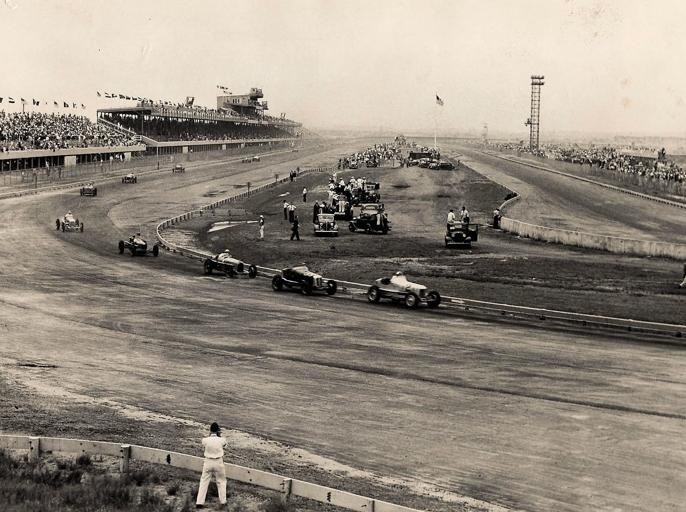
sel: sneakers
[196,504,203,509]
[222,503,227,506]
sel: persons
[493,207,503,229]
[258,214,264,239]
[65,209,77,220]
[499,142,686,194]
[390,271,410,289]
[194,422,229,508]
[0,97,293,183]
[133,232,147,245]
[85,183,94,189]
[217,249,233,261]
[282,135,471,241]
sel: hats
[210,422,218,432]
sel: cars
[272,266,336,297]
[80,187,97,197]
[242,155,260,163]
[122,176,137,184]
[56,218,83,233]
[411,157,454,169]
[172,163,185,172]
[445,220,478,246]
[311,182,391,236]
[204,249,256,279]
[119,235,158,258]
[368,276,441,309]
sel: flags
[435,93,444,106]
[96,90,195,107]
